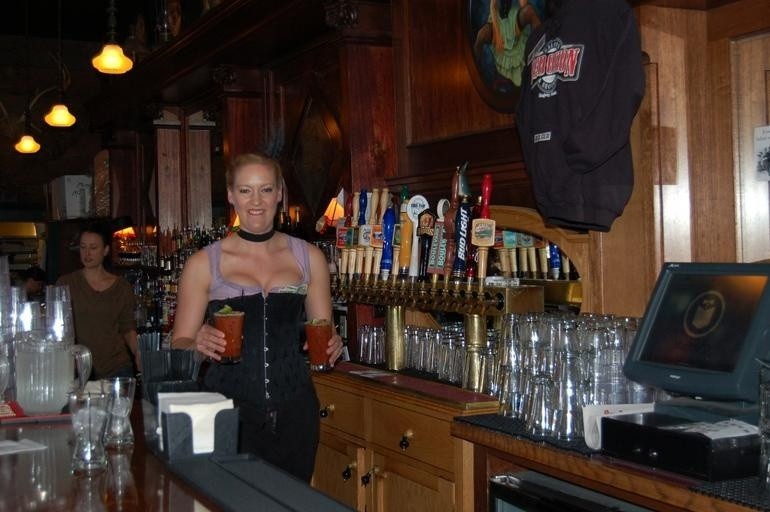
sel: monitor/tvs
[621,262,770,426]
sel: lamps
[13,0,134,155]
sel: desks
[451,413,769,510]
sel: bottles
[283,205,303,234]
[118,223,228,331]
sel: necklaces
[237,229,275,242]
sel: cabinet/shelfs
[371,380,462,510]
[310,372,371,510]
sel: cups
[108,419,135,447]
[212,312,245,364]
[102,376,137,418]
[70,441,109,477]
[70,393,112,439]
[303,319,334,373]
[355,310,643,441]
[0,256,93,416]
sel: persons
[170,152,344,485]
[21,267,46,301]
[56,223,143,381]
[474,0,542,88]
